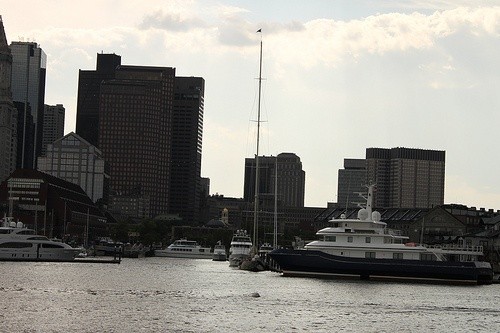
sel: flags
[257,29,261,32]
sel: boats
[152,238,213,259]
[228,228,253,268]
[258,243,274,257]
[267,180,492,285]
[212,241,226,261]
[1,213,83,261]
